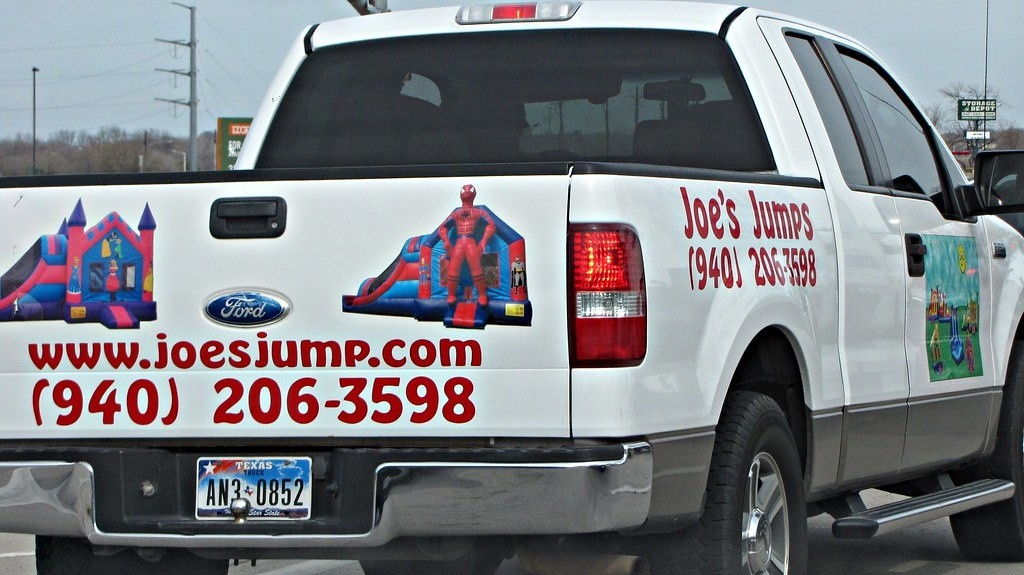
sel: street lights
[32,66,40,176]
[171,149,186,173]
[138,140,173,173]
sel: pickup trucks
[0,2,1024,575]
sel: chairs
[634,113,731,168]
